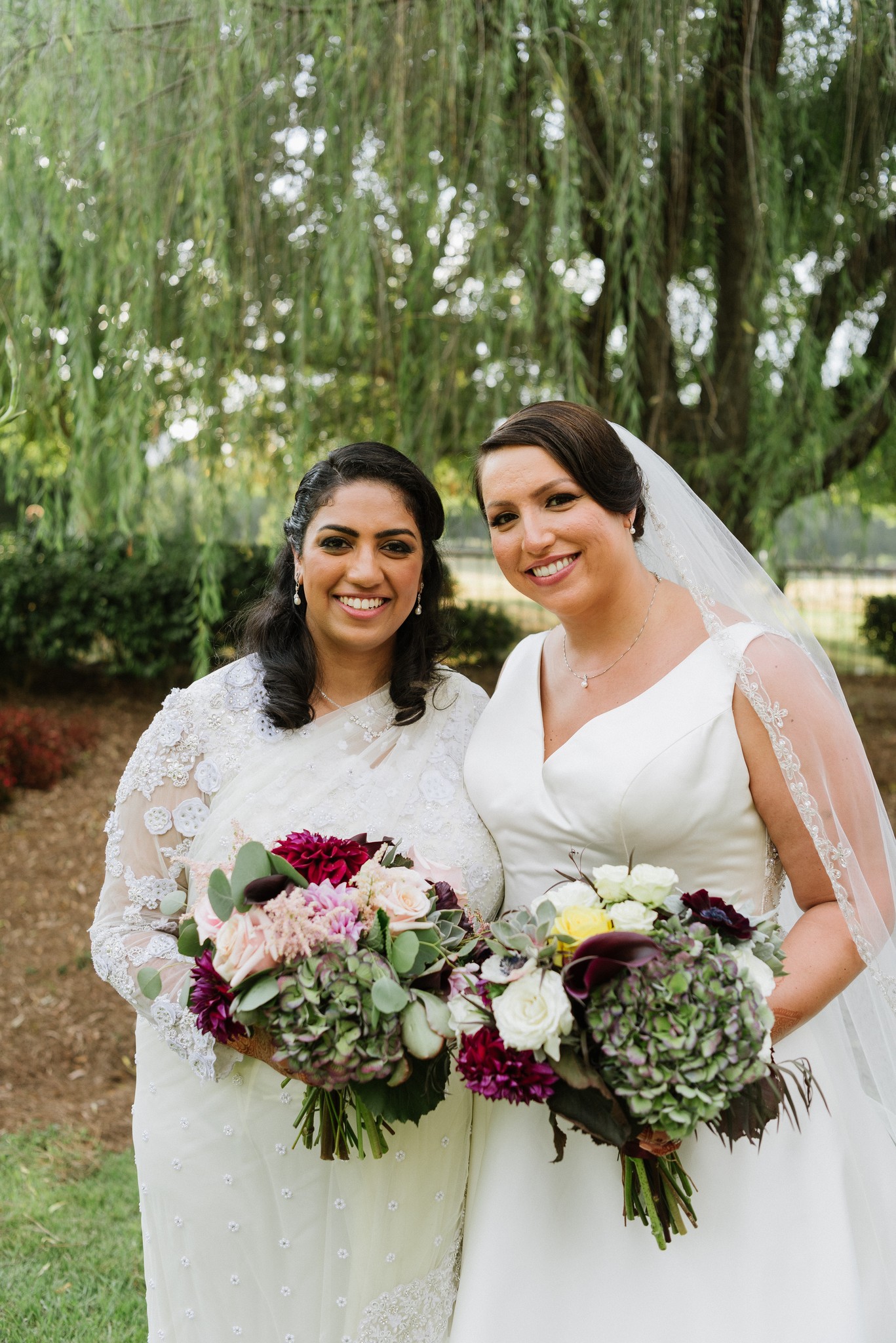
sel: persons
[85,442,504,1343]
[460,400,896,1343]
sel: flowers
[447,847,831,1250]
[136,829,478,1160]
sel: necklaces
[563,572,662,688]
[315,683,395,743]
